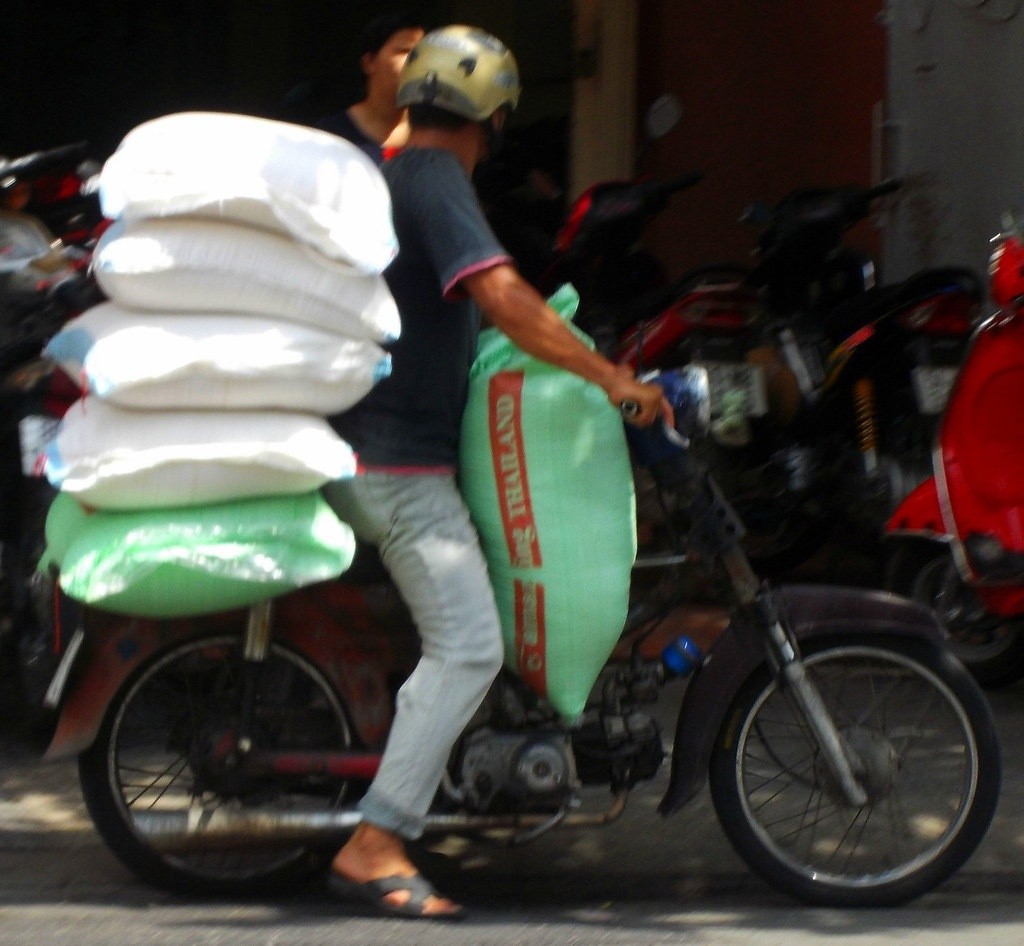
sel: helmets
[394,22,522,124]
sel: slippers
[321,859,463,923]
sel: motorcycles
[43,364,1004,917]
[744,172,988,602]
[545,163,889,604]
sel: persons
[320,24,675,920]
[315,1,448,164]
